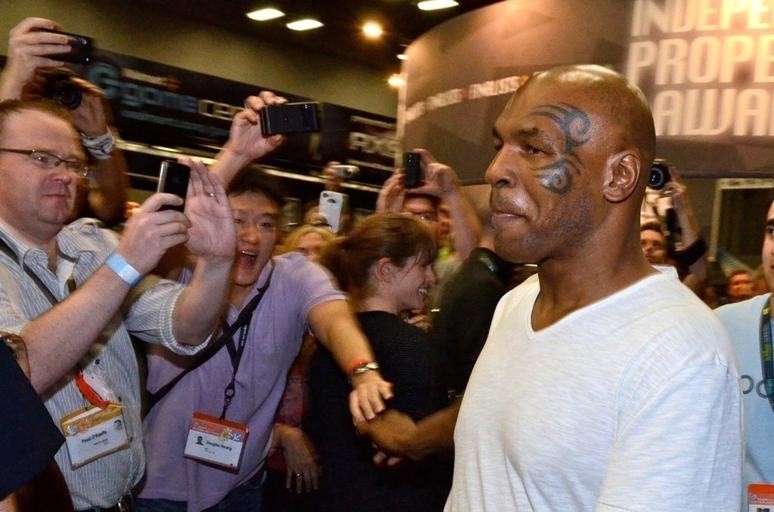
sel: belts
[85,495,131,511]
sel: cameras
[32,69,83,110]
[647,163,670,190]
[332,164,360,179]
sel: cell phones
[156,161,191,211]
[317,189,348,234]
[260,100,325,137]
[28,26,94,67]
[401,151,421,189]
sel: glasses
[2,147,89,178]
[283,224,332,233]
[403,210,436,221]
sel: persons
[1,11,774,511]
